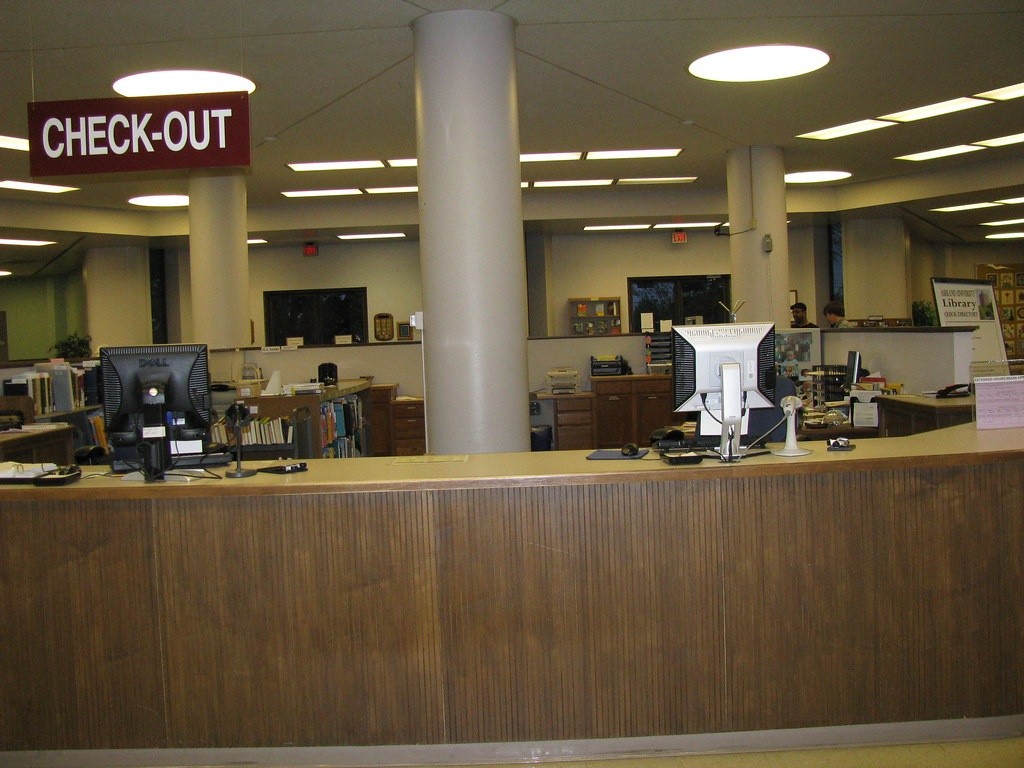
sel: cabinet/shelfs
[390,396,426,457]
[587,375,686,449]
[227,406,311,459]
[0,395,103,423]
[234,378,399,459]
[568,297,622,336]
[537,390,594,450]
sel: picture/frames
[397,322,413,340]
[986,273,999,290]
[789,290,798,323]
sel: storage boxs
[812,364,870,407]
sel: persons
[774,343,801,380]
[790,299,852,329]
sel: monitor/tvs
[843,351,871,390]
[672,321,777,460]
[100,344,212,480]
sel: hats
[791,303,806,311]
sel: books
[210,415,295,444]
[6,357,111,454]
[318,395,367,458]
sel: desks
[797,422,875,440]
[0,421,74,463]
[869,393,977,438]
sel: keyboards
[652,435,767,453]
[110,453,232,472]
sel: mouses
[621,443,638,455]
[59,463,80,475]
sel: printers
[546,368,581,395]
